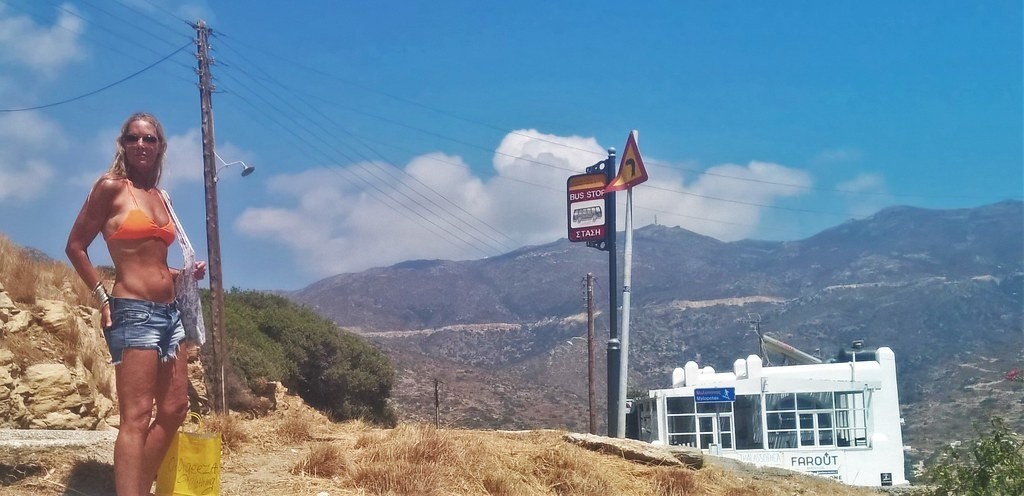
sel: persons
[64,113,206,496]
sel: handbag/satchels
[160,189,206,347]
[155,411,221,496]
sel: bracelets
[92,281,108,310]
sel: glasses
[124,135,159,142]
